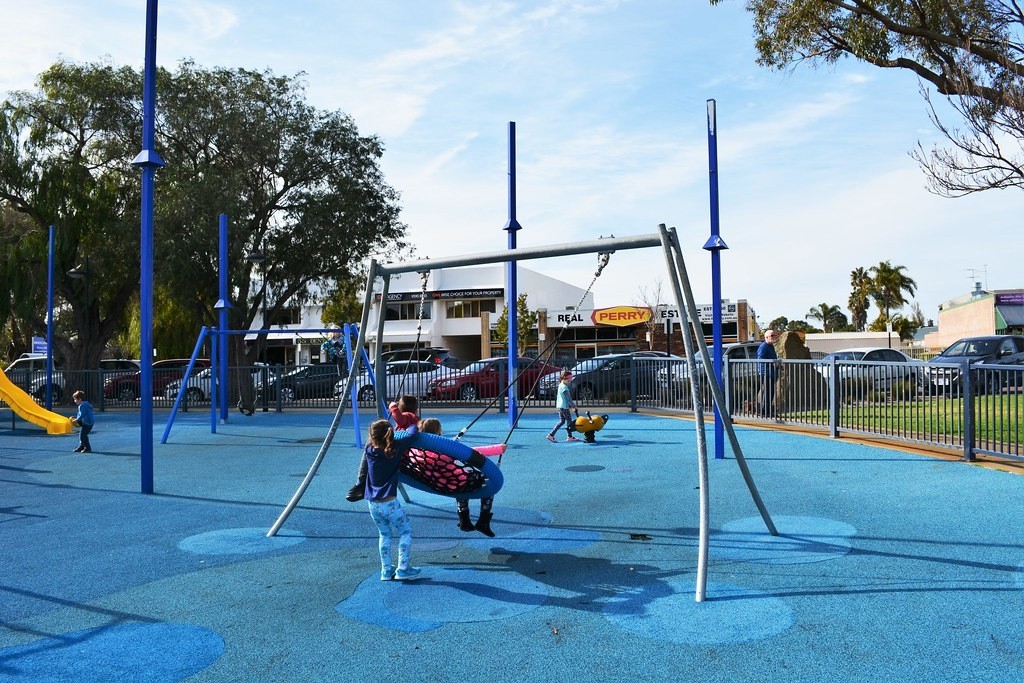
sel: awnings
[996,305,1024,330]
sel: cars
[254,362,348,401]
[809,351,829,364]
[919,335,1024,395]
[103,359,211,401]
[333,360,461,402]
[3,356,48,385]
[813,347,923,391]
[538,353,665,400]
[165,361,273,402]
[428,357,564,401]
[610,350,687,369]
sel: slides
[0,367,77,435]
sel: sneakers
[381,565,396,580]
[547,434,557,442]
[566,437,579,441]
[396,565,421,578]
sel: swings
[386,253,611,500]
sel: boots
[458,508,476,531]
[475,509,495,537]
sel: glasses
[767,335,774,337]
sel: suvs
[29,358,142,403]
[373,347,457,368]
[656,340,764,392]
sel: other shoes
[345,485,364,501]
[81,447,91,453]
[73,446,83,452]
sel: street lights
[67,254,91,402]
[245,238,271,412]
[883,291,891,348]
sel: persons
[346,396,420,502]
[410,418,507,538]
[365,420,421,582]
[320,325,347,371]
[73,391,94,453]
[546,371,579,442]
[757,330,778,417]
